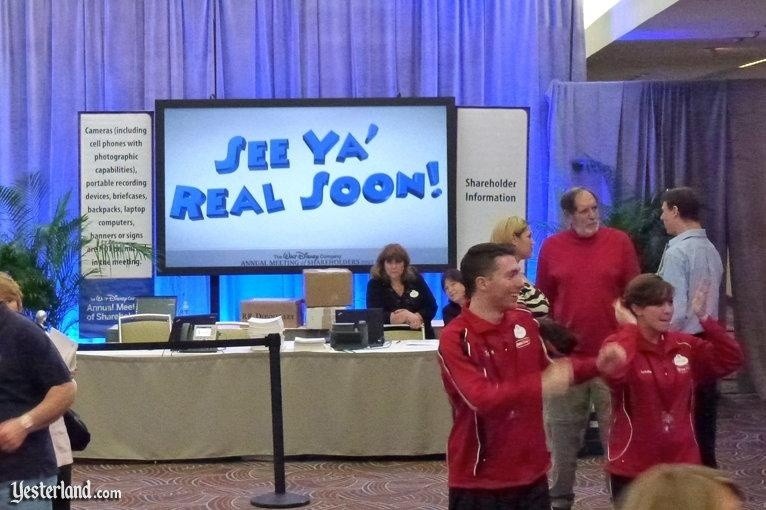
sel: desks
[105,324,119,341]
[72,340,454,465]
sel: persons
[655,186,724,470]
[0,299,77,510]
[613,462,750,510]
[435,243,626,510]
[599,272,745,504]
[441,269,466,326]
[0,271,90,510]
[490,216,551,323]
[535,186,643,510]
[366,244,438,339]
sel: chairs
[383,323,425,340]
[283,327,332,342]
[214,321,252,339]
[118,313,173,343]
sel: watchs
[21,415,34,433]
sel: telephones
[178,323,217,348]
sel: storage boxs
[301,303,347,330]
[240,297,303,328]
[302,267,354,307]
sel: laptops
[135,296,177,321]
[335,308,384,347]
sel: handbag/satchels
[59,404,94,454]
